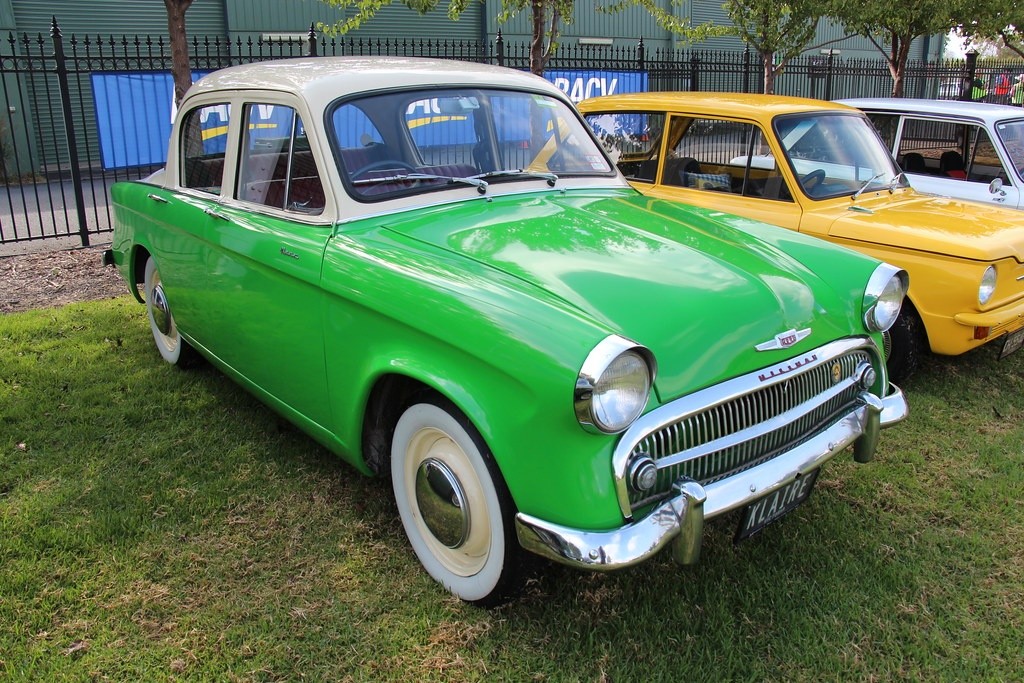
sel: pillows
[684,172,733,193]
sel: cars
[114,59,910,606]
[725,91,1020,305]
[579,92,1021,365]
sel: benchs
[248,164,479,209]
[616,156,704,182]
[196,144,388,189]
[680,171,790,200]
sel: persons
[972,69,989,99]
[1009,74,1024,104]
[993,66,1011,95]
[940,151,971,179]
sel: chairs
[941,150,969,180]
[904,153,937,176]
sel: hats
[1015,74,1024,82]
